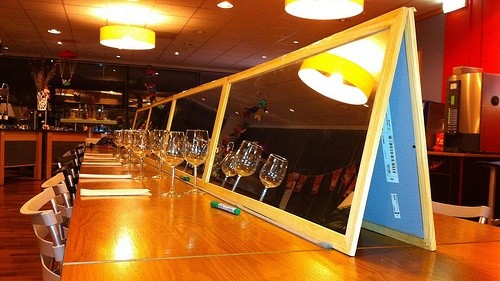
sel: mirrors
[129,7,438,256]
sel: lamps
[99,26,156,51]
[284,0,365,20]
[297,54,374,105]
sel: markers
[208,201,241,216]
[180,173,193,182]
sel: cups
[203,140,225,169]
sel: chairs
[432,201,491,225]
[19,144,86,281]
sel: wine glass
[161,131,187,198]
[113,130,125,162]
[231,140,262,191]
[182,129,210,194]
[221,149,237,188]
[149,130,167,179]
[122,130,137,166]
[259,155,288,202]
[133,130,151,182]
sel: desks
[41,129,105,180]
[0,127,45,185]
[60,117,116,137]
[61,146,500,281]
